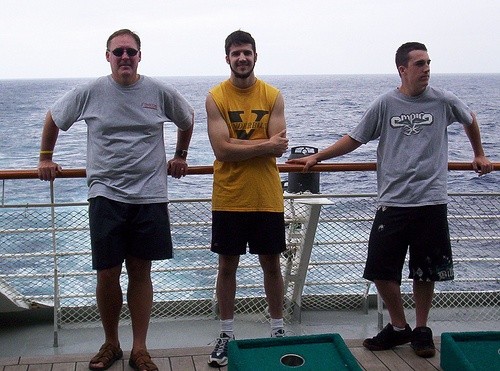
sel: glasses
[107,47,139,56]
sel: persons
[284,41,495,357]
[38,27,195,371]
[204,27,289,367]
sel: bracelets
[40,151,54,153]
[175,148,188,156]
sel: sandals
[129,349,158,371]
[89,340,123,371]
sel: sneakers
[363,323,412,351]
[271,329,286,337]
[207,333,235,368]
[412,326,435,358]
